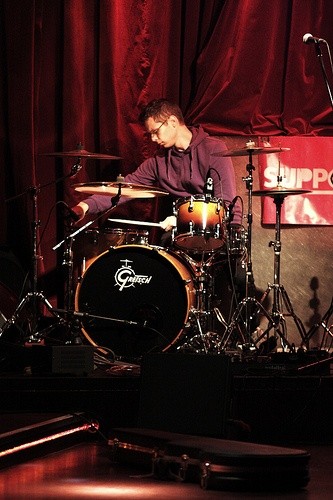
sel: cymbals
[210,146,292,159]
[245,185,310,197]
[40,150,121,159]
[73,182,169,198]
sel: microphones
[302,33,326,44]
[141,316,152,328]
[228,196,238,213]
[203,170,211,194]
[63,202,80,222]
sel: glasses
[146,118,170,137]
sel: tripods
[173,150,311,356]
[0,157,122,346]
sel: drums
[94,228,149,257]
[200,226,245,262]
[64,226,100,257]
[175,193,227,250]
[75,246,205,361]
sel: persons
[65,99,257,324]
